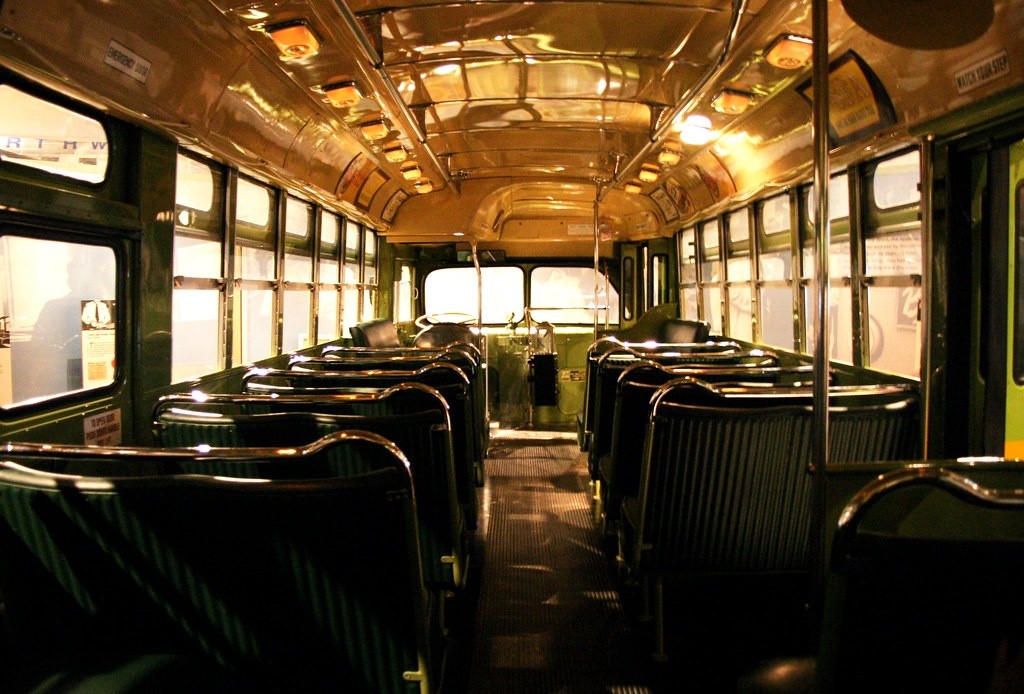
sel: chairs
[413,323,476,347]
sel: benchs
[616,378,917,661]
[0,429,447,694]
[593,347,766,553]
[745,463,1024,694]
[322,341,489,488]
[607,361,840,598]
[350,319,401,350]
[585,337,740,531]
[239,362,480,572]
[284,349,487,547]
[150,381,472,676]
[576,318,711,451]
